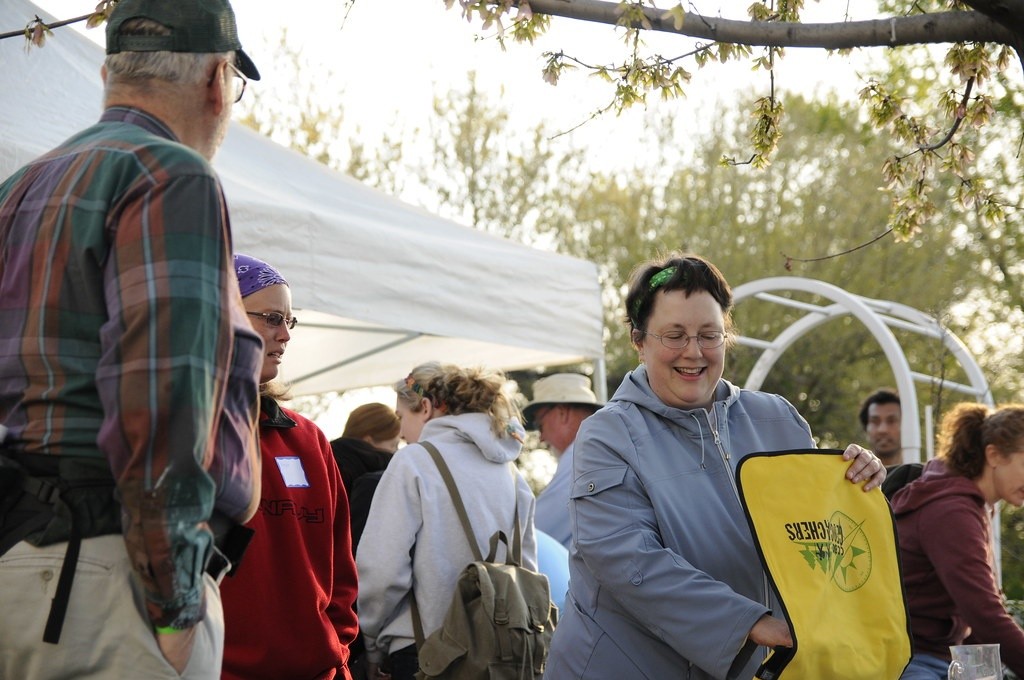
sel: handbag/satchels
[735,450,913,680]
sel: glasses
[530,405,556,429]
[207,61,246,103]
[645,329,727,349]
[247,310,296,329]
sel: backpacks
[407,441,560,680]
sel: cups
[948,644,1004,680]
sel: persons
[354,358,538,680]
[1,0,260,680]
[219,253,359,680]
[542,254,886,680]
[888,403,1024,680]
[521,373,606,551]
[329,403,401,561]
[859,391,903,464]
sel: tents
[1,0,607,439]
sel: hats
[106,0,258,80]
[523,374,605,430]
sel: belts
[206,546,229,583]
